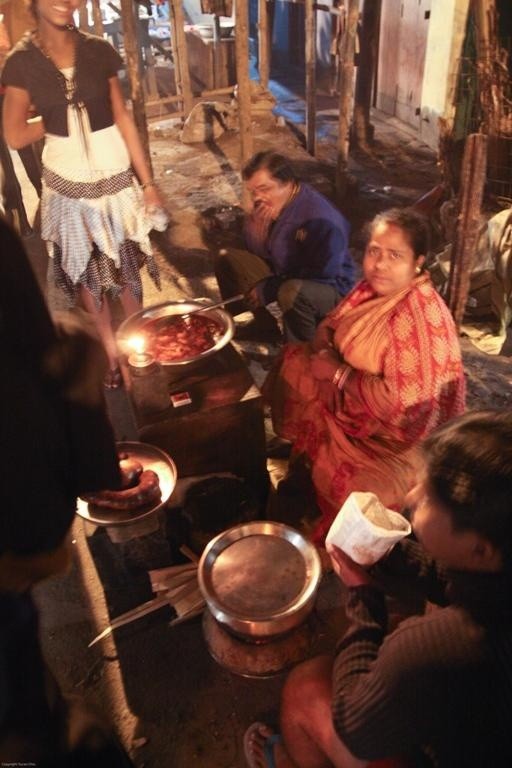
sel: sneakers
[232,317,282,343]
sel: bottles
[126,353,173,412]
[148,203,167,233]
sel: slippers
[242,722,282,768]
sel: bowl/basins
[116,299,235,376]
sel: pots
[195,520,324,638]
[200,606,323,680]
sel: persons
[243,406,512,767]
[268,200,469,529]
[232,145,361,353]
[0,331,152,767]
[0,0,166,398]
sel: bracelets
[135,176,164,193]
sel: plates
[75,440,177,528]
